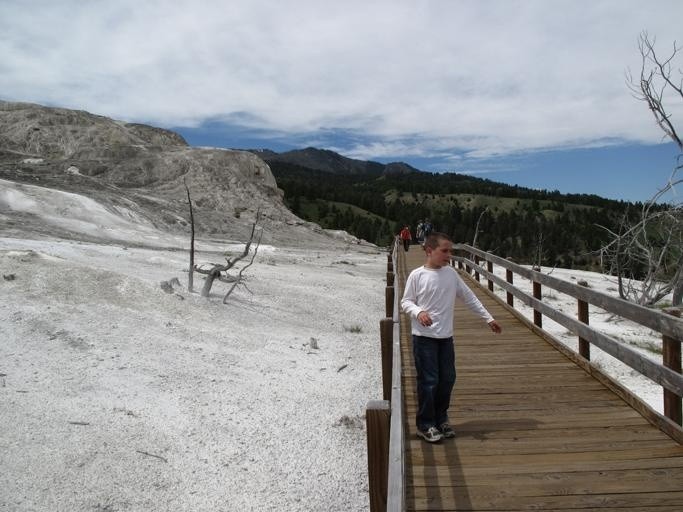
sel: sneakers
[416,423,456,443]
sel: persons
[400,231,503,443]
[400,217,434,252]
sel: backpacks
[425,224,432,233]
